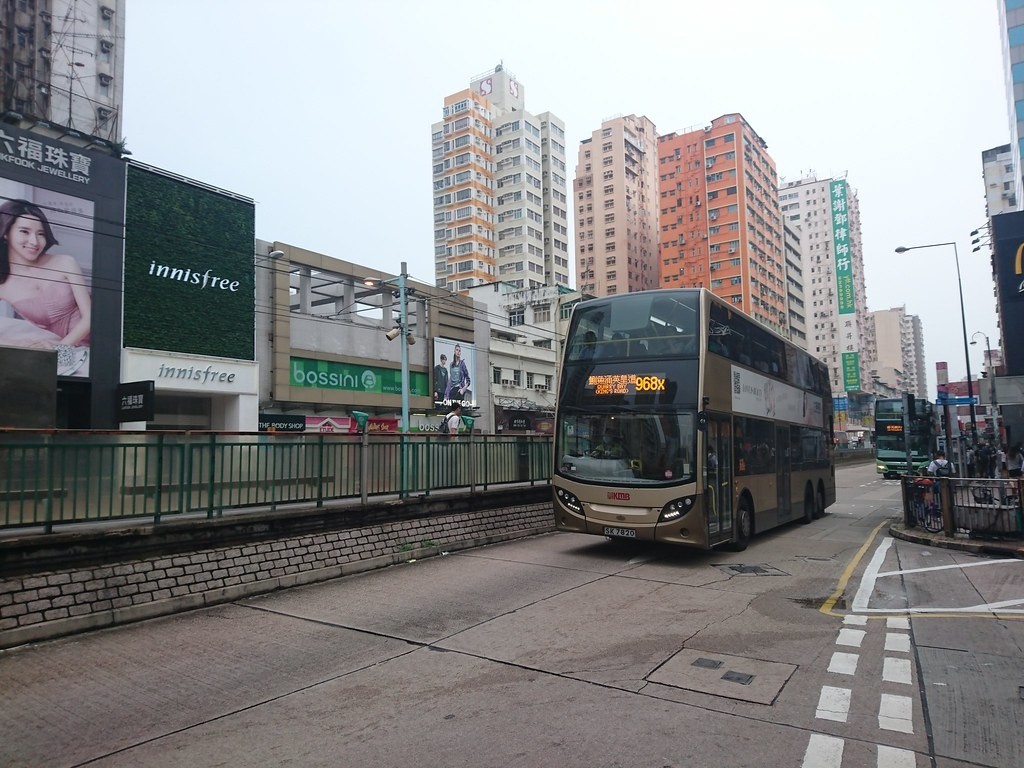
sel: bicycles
[971,481,1020,506]
[909,482,944,533]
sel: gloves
[605,451,611,456]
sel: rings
[50,344,89,376]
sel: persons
[926,440,1024,505]
[433,344,471,403]
[575,326,771,463]
[0,199,91,379]
[442,402,462,448]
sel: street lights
[970,331,994,377]
[363,276,409,498]
[895,242,979,445]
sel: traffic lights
[858,438,861,440]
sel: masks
[602,436,611,443]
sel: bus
[874,399,942,479]
[552,288,836,553]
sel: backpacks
[978,449,988,463]
[435,415,457,445]
[933,460,951,477]
